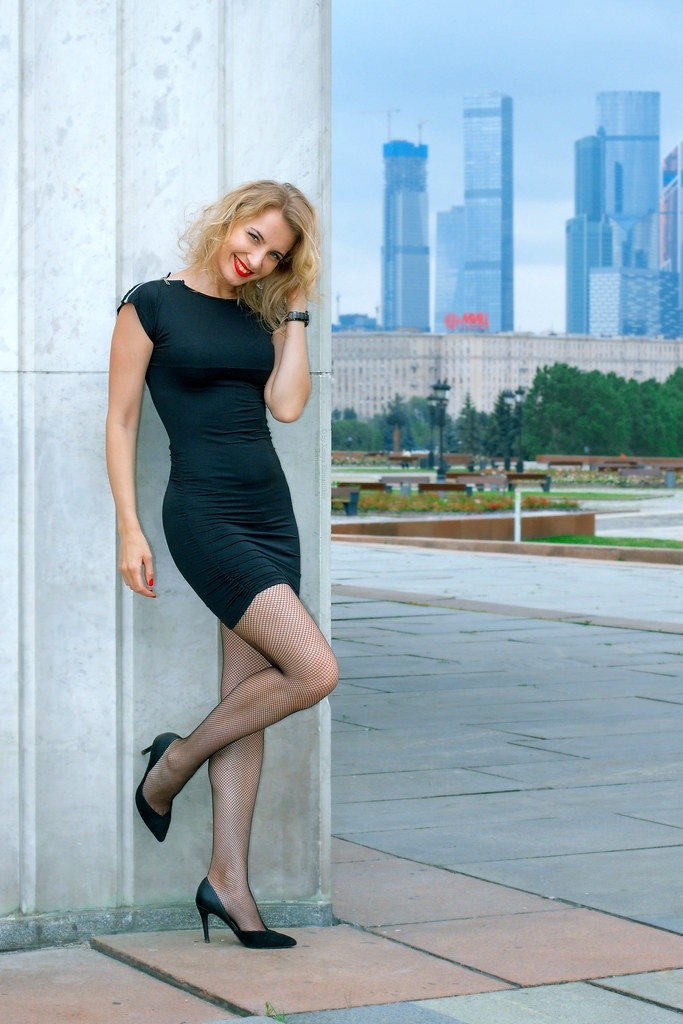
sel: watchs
[286,310,310,327]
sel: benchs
[417,482,473,497]
[379,475,430,496]
[444,453,476,472]
[547,459,584,469]
[491,456,519,469]
[387,457,419,470]
[616,468,662,489]
[330,486,359,516]
[336,482,393,494]
[445,471,485,492]
[412,454,428,469]
[456,475,507,492]
[505,473,551,491]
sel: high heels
[136,733,182,843]
[195,876,300,949]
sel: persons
[105,182,340,949]
